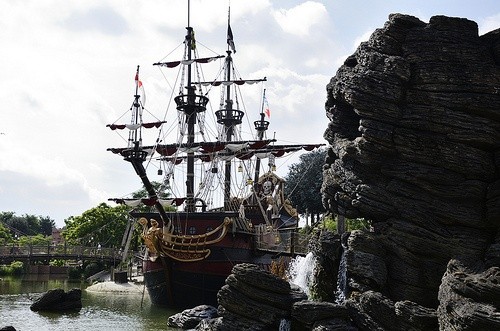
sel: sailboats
[106,0,327,307]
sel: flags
[263,92,271,118]
[227,10,237,54]
[134,70,146,108]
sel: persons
[97,242,102,254]
